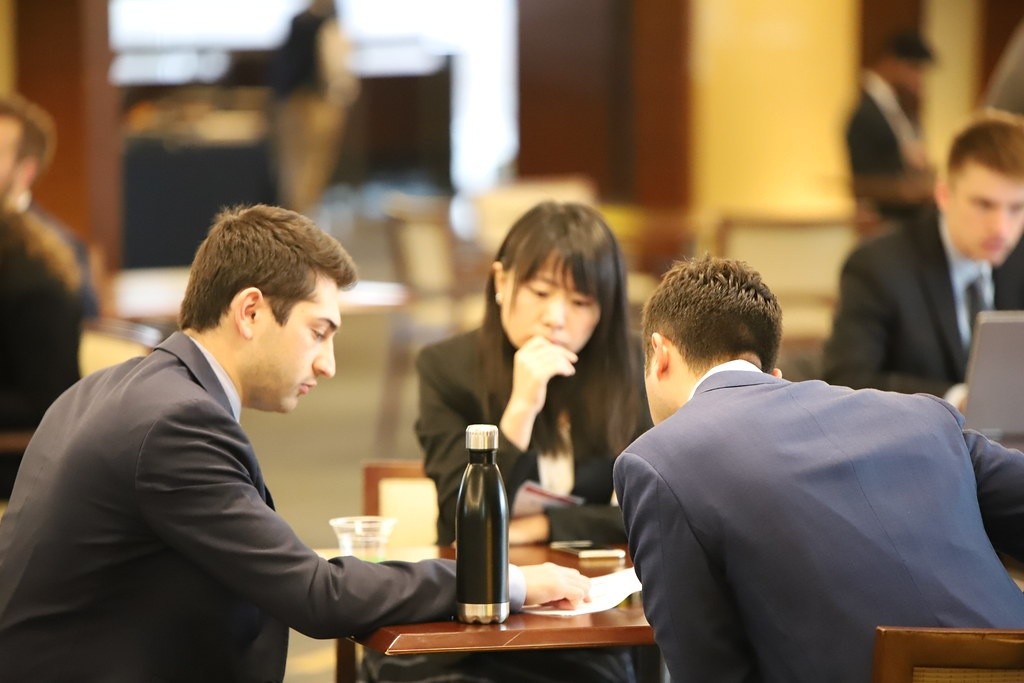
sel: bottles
[456,424,510,625]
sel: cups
[330,517,396,561]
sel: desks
[124,134,270,268]
[317,544,655,683]
[462,290,833,380]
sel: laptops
[965,310,1024,440]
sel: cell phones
[551,541,625,558]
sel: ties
[960,272,993,335]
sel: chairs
[364,462,439,542]
[719,218,864,294]
[79,314,160,381]
[873,625,1024,683]
[479,179,594,274]
[379,194,476,464]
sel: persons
[0,93,103,515]
[269,1,358,219]
[823,111,1024,455]
[614,257,1024,683]
[843,34,944,212]
[414,200,654,547]
[0,205,591,683]
